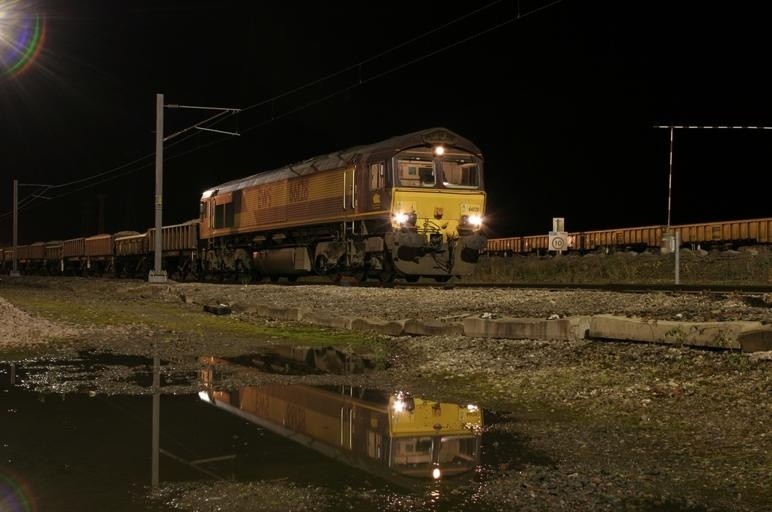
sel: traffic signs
[548,231,568,251]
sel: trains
[181,350,486,492]
[0,121,488,283]
[484,217,772,258]
